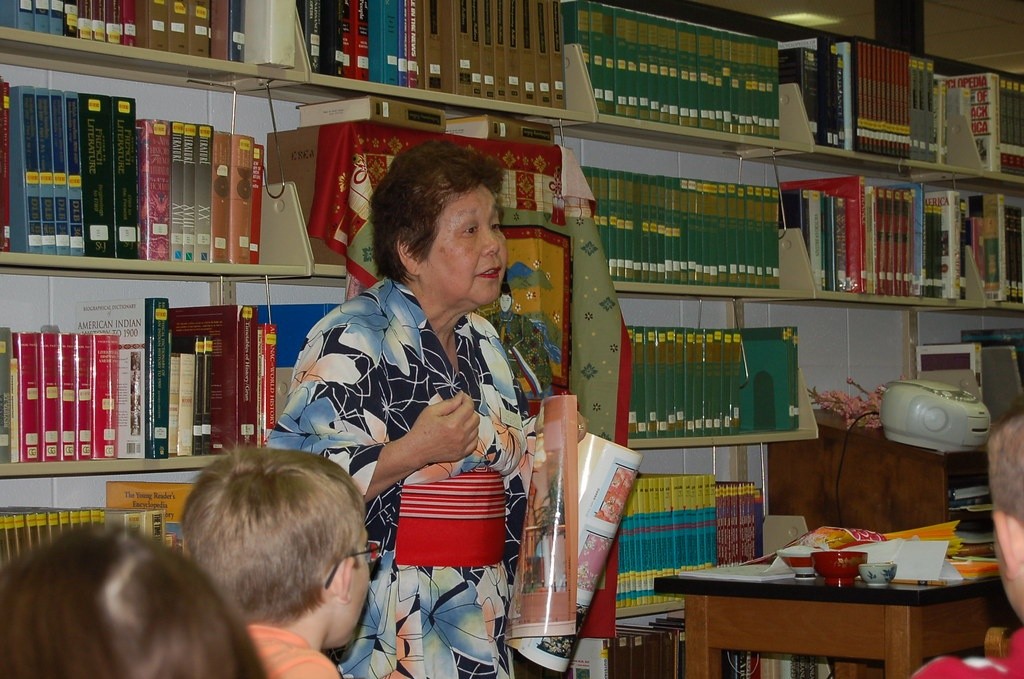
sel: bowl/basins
[777,550,818,580]
[859,564,897,588]
[812,551,867,585]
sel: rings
[577,424,583,430]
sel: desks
[654,570,1024,679]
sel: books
[0,0,1024,679]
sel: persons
[267,139,589,679]
[180,446,370,679]
[911,415,1024,679]
[0,522,266,679]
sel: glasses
[324,539,384,589]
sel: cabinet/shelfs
[0,0,1024,626]
